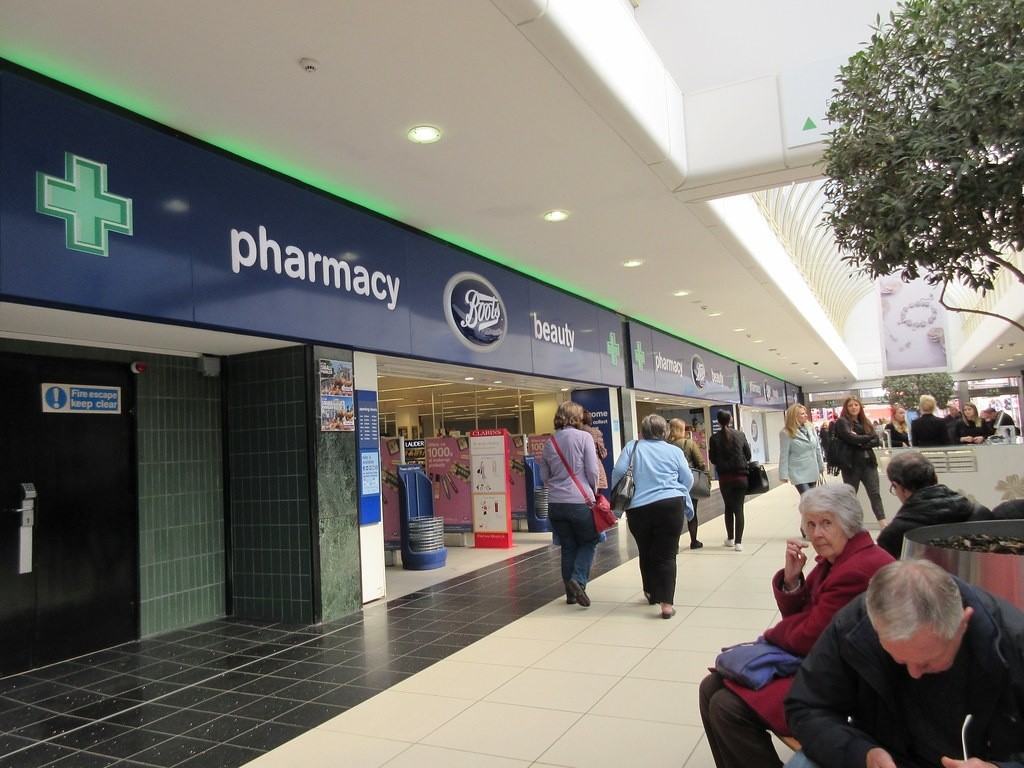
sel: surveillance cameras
[997,344,1004,349]
[1009,343,1015,347]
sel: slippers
[663,608,676,619]
[649,597,660,604]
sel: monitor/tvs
[993,411,1005,428]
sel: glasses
[890,484,905,496]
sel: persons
[611,414,695,618]
[538,401,609,607]
[437,429,442,437]
[698,482,898,768]
[816,412,841,476]
[878,451,994,558]
[783,558,1024,768]
[778,403,825,539]
[871,395,1020,448]
[667,418,707,548]
[709,409,752,551]
[834,395,888,532]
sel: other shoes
[568,580,590,607]
[567,597,578,604]
[691,541,703,549]
[724,539,734,547]
[735,543,741,551]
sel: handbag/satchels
[715,636,805,691]
[831,419,854,470]
[745,461,769,494]
[611,440,639,512]
[591,493,616,533]
[689,467,710,499]
